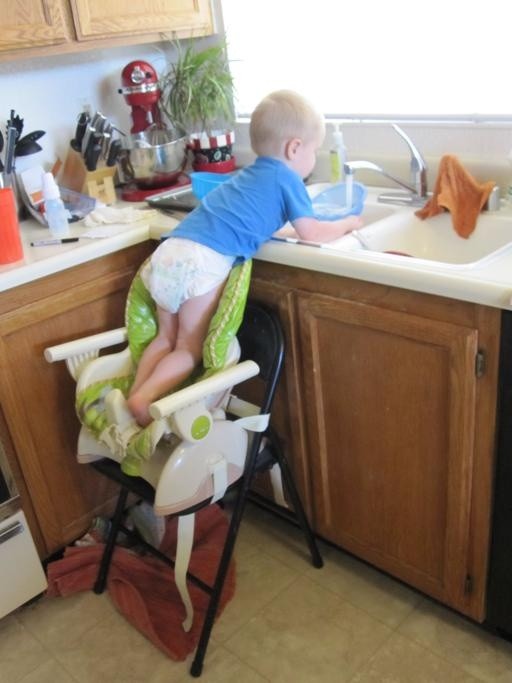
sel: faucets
[342,123,431,201]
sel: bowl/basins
[188,168,235,199]
[308,180,367,218]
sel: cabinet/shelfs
[1,240,153,563]
[244,259,502,625]
[0,0,219,58]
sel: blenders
[111,59,196,203]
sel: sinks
[280,182,403,243]
[336,208,512,270]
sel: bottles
[40,171,71,237]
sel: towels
[416,154,495,238]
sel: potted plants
[158,26,240,172]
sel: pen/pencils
[31,238,79,247]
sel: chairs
[90,297,327,677]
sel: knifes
[75,105,121,169]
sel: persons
[125,88,363,433]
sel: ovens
[1,439,54,629]
